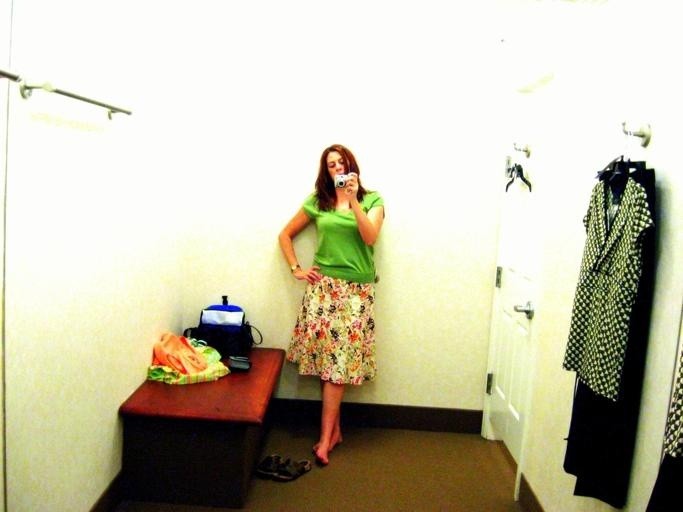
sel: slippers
[254,454,284,476]
[272,458,311,480]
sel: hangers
[598,153,649,189]
[506,144,532,193]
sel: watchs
[291,265,300,272]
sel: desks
[119,347,286,507]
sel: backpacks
[183,296,263,360]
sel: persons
[278,144,385,465]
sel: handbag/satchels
[152,332,207,375]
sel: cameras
[335,174,350,188]
